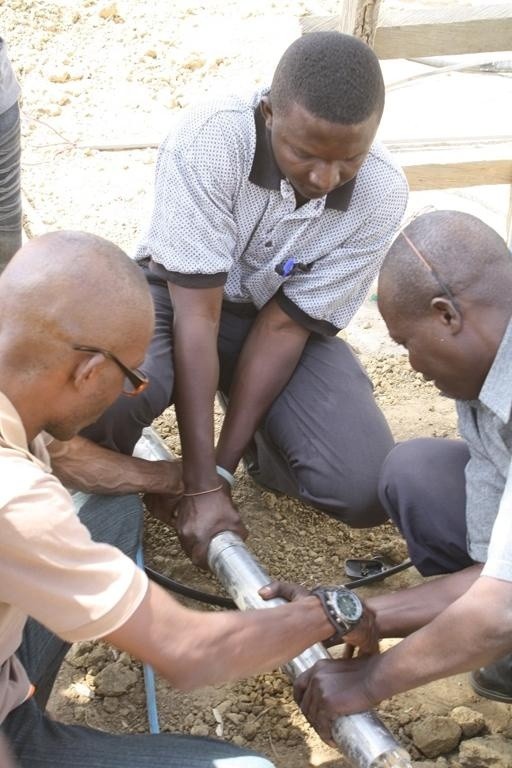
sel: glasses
[73,345,150,397]
[391,203,466,318]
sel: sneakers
[216,388,282,498]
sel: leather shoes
[469,648,512,704]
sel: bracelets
[214,465,237,488]
[181,484,224,499]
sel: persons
[0,36,25,274]
[77,28,409,529]
[257,209,512,747]
[0,228,380,768]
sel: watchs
[312,583,363,648]
